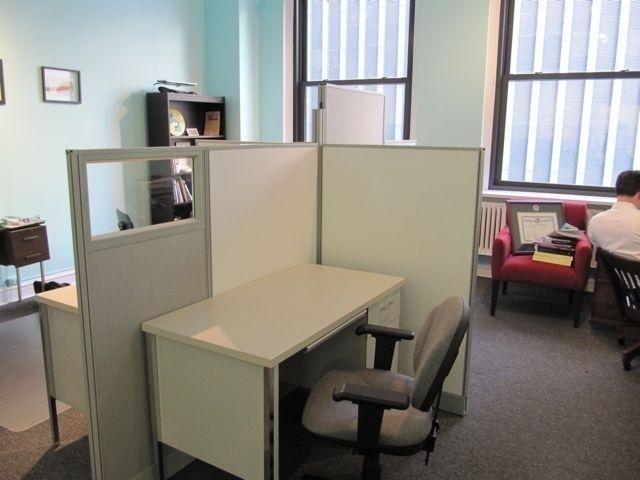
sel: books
[175,109,220,211]
[534,221,581,268]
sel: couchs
[490,200,591,327]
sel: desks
[37,284,87,446]
[141,262,407,480]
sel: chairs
[598,248,639,371]
[302,296,472,480]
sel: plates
[170,109,185,136]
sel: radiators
[479,202,606,266]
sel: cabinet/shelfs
[147,93,226,224]
[0,218,51,302]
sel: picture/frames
[0,59,6,105]
[507,200,565,256]
[42,66,81,104]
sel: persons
[587,170,640,308]
